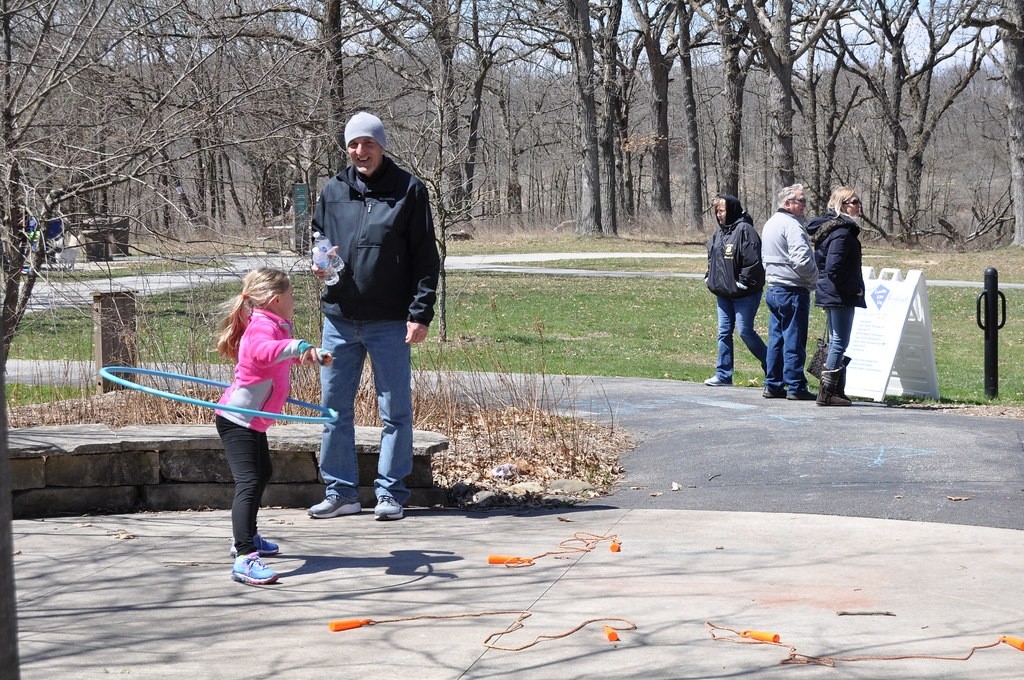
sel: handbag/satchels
[806,338,852,380]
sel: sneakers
[786,389,817,400]
[763,386,788,397]
[231,553,278,584]
[375,495,403,520]
[230,533,279,554]
[705,374,733,386]
[308,494,361,518]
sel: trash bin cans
[83,217,113,261]
[109,216,131,256]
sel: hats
[344,112,386,149]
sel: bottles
[312,231,345,272]
[312,247,339,286]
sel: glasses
[844,201,862,205]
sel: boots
[837,371,851,401]
[816,363,851,405]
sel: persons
[216,268,333,585]
[806,187,866,406]
[308,111,440,520]
[704,195,768,387]
[760,183,817,400]
